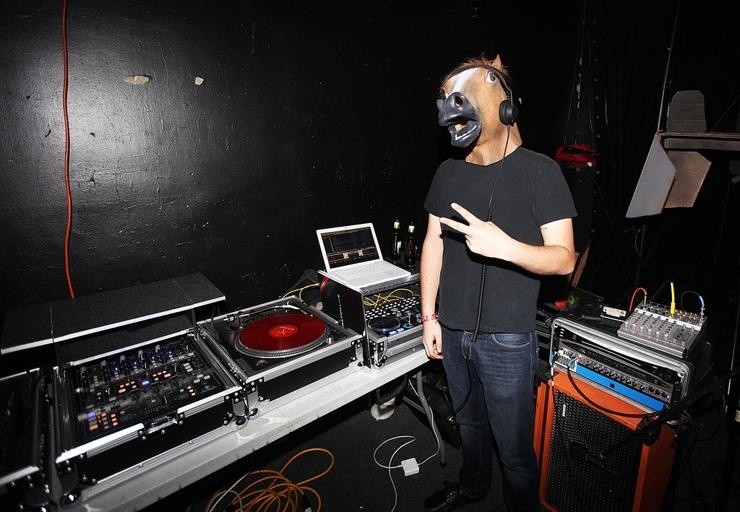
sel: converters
[401,457,420,477]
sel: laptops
[316,222,412,289]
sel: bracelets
[419,314,439,323]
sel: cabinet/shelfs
[0,270,227,380]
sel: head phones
[441,64,520,127]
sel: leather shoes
[423,481,489,512]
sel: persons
[416,54,578,511]
